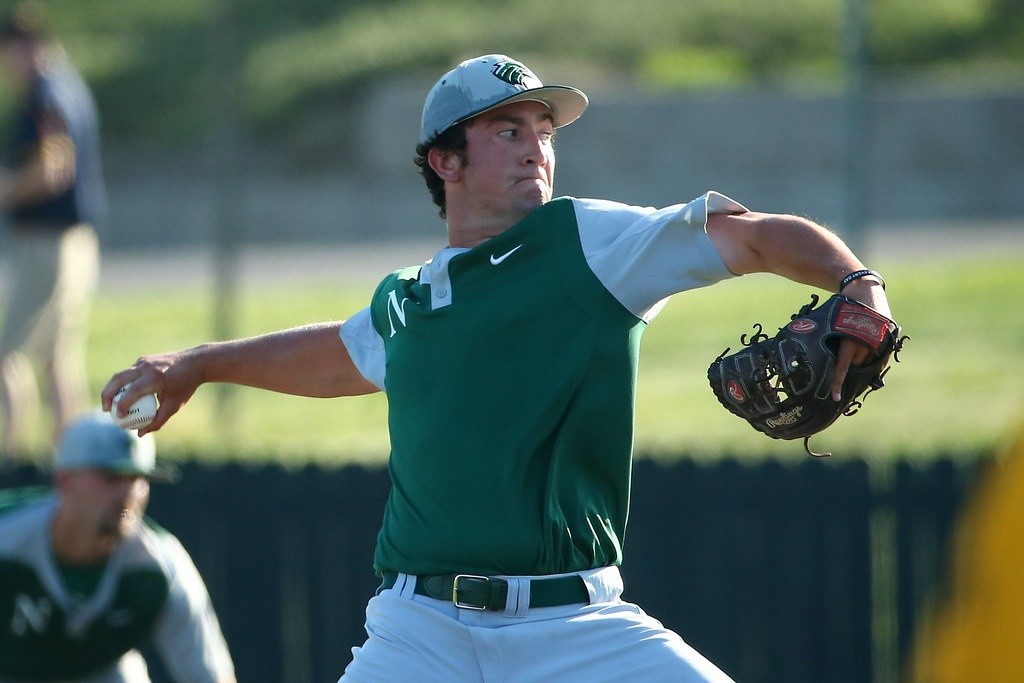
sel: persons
[102,54,901,683]
[0,406,237,683]
[0,0,112,459]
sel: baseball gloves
[705,294,901,441]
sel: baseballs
[110,381,157,429]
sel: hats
[58,410,157,476]
[420,54,588,143]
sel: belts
[374,572,590,611]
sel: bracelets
[840,269,886,292]
